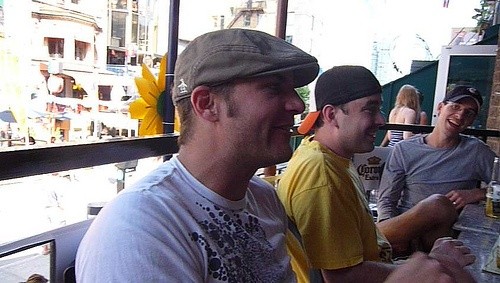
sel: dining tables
[454,202,500,283]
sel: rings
[452,201,457,206]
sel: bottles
[484,156,500,220]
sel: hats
[298,65,382,136]
[444,85,483,115]
[171,28,319,103]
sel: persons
[75,28,456,283]
[379,91,428,149]
[373,84,496,260]
[385,84,421,149]
[276,65,476,283]
